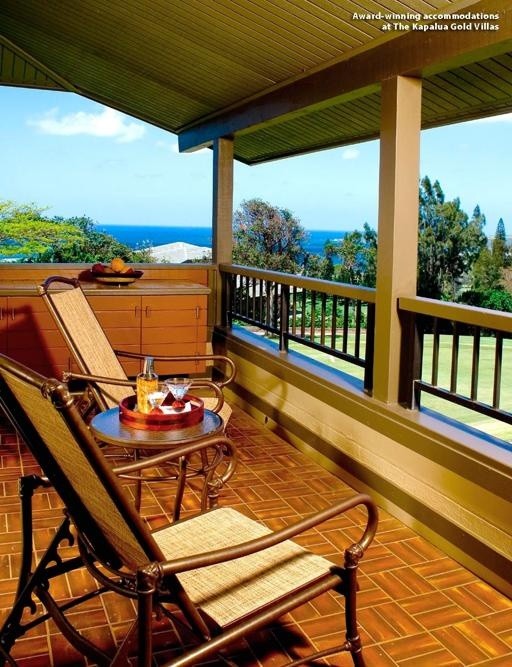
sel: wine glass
[163,378,193,409]
[147,390,168,415]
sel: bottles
[137,357,158,413]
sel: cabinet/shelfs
[0,279,212,418]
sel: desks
[90,408,224,522]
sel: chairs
[37,276,237,468]
[0,354,379,667]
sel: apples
[92,258,133,274]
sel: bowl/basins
[91,272,144,286]
